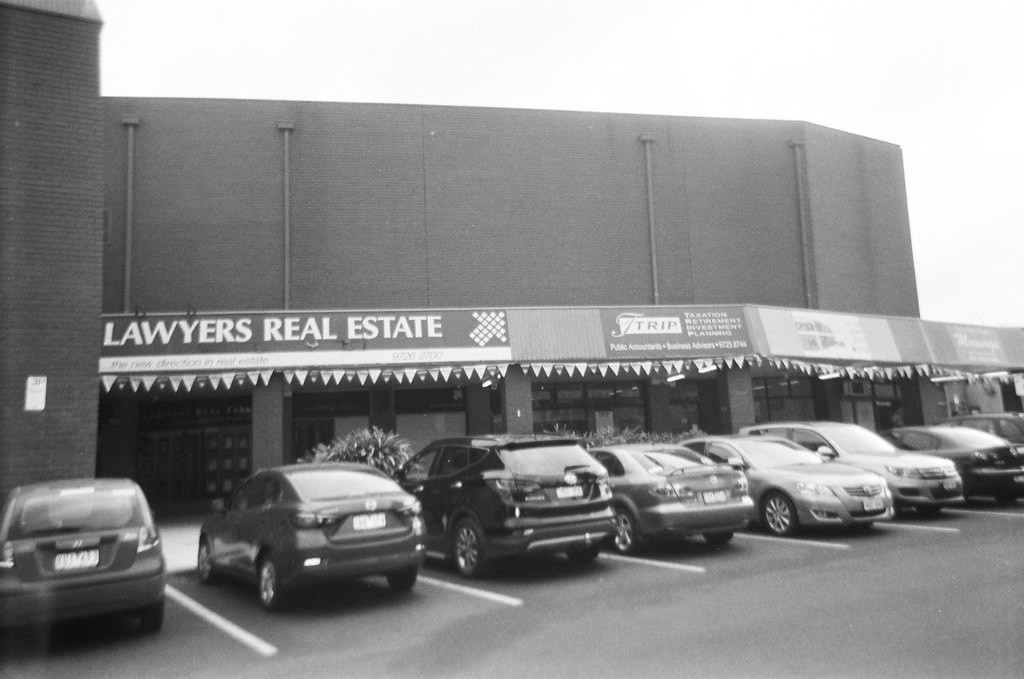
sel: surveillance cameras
[491,384,497,390]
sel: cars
[587,443,754,555]
[881,427,1024,502]
[0,478,165,633]
[676,436,894,537]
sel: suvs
[390,434,618,579]
[939,414,1024,446]
[738,423,966,516]
[198,462,427,612]
